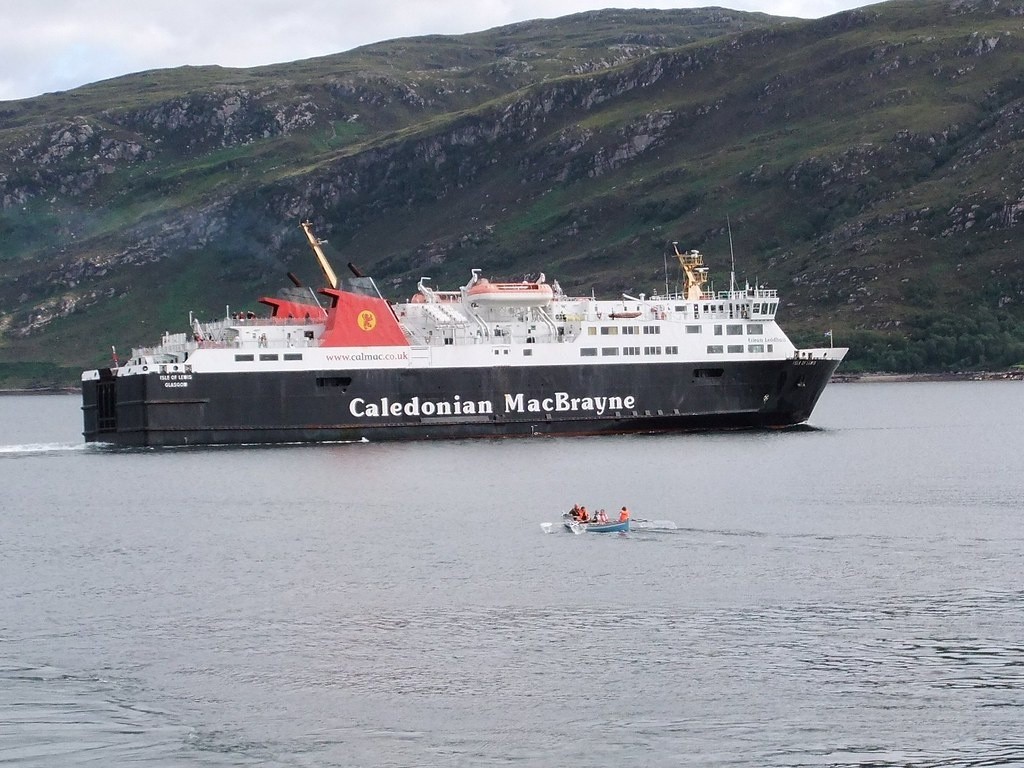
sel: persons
[231,312,238,325]
[192,333,268,349]
[598,509,609,523]
[616,506,630,523]
[247,310,256,326]
[239,311,246,325]
[591,510,602,523]
[569,503,581,521]
[578,506,590,523]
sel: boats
[559,511,631,534]
[78,216,850,449]
[409,288,463,304]
[465,278,554,308]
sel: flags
[823,330,831,337]
